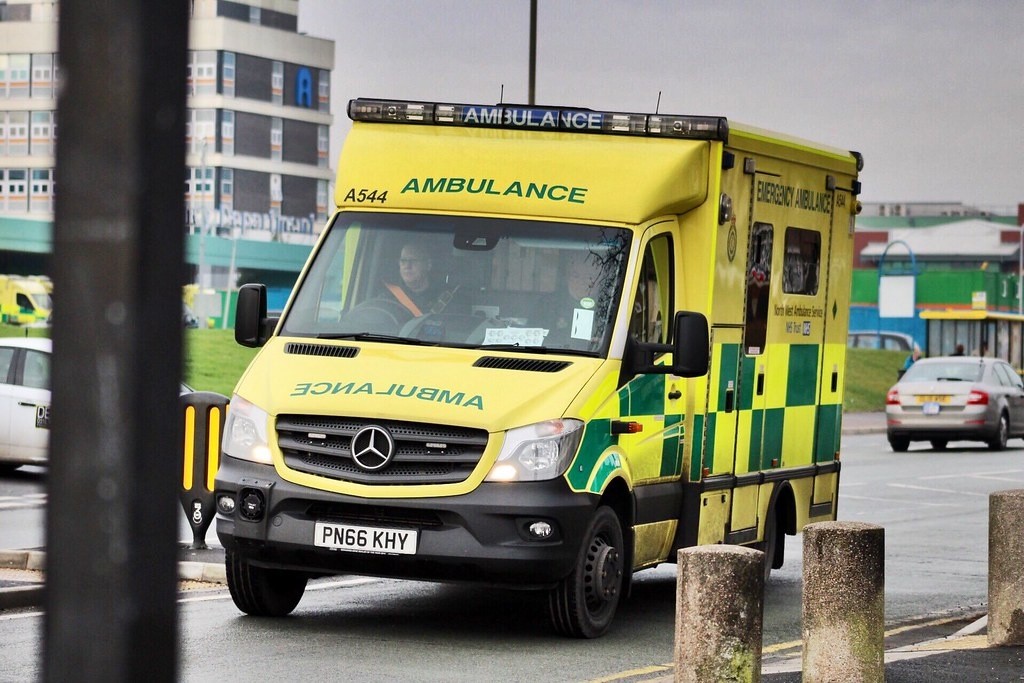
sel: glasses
[398,258,423,266]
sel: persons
[902,346,922,371]
[377,238,457,342]
[553,245,611,342]
[949,344,966,356]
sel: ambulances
[214,97,866,640]
[0,274,53,325]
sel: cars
[1,336,200,473]
[885,355,1024,453]
